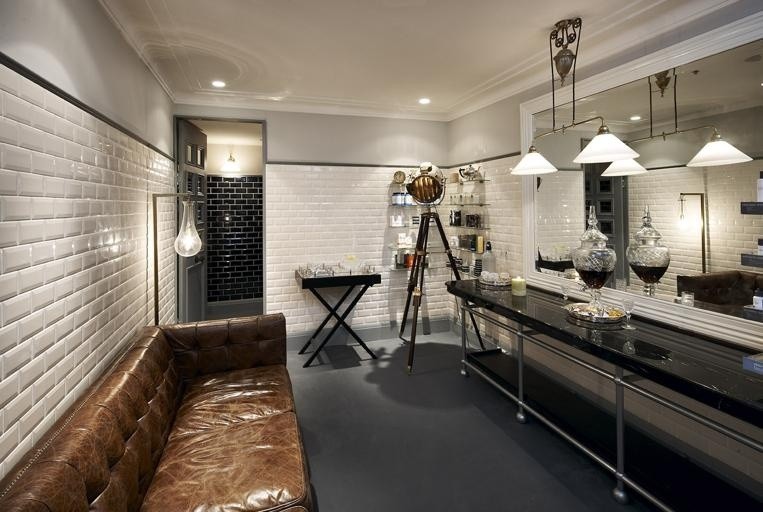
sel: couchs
[0,311,314,512]
[676,269,762,312]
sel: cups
[481,271,510,283]
[681,292,695,306]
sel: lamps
[509,17,640,177]
[150,193,202,326]
[598,67,753,179]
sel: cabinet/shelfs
[387,178,492,279]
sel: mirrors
[517,11,762,353]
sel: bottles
[392,192,413,206]
[482,241,496,273]
[753,290,763,311]
[573,205,619,316]
[624,204,671,299]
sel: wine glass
[622,329,638,354]
[621,298,636,329]
[559,282,571,307]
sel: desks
[294,267,381,368]
[445,278,763,511]
